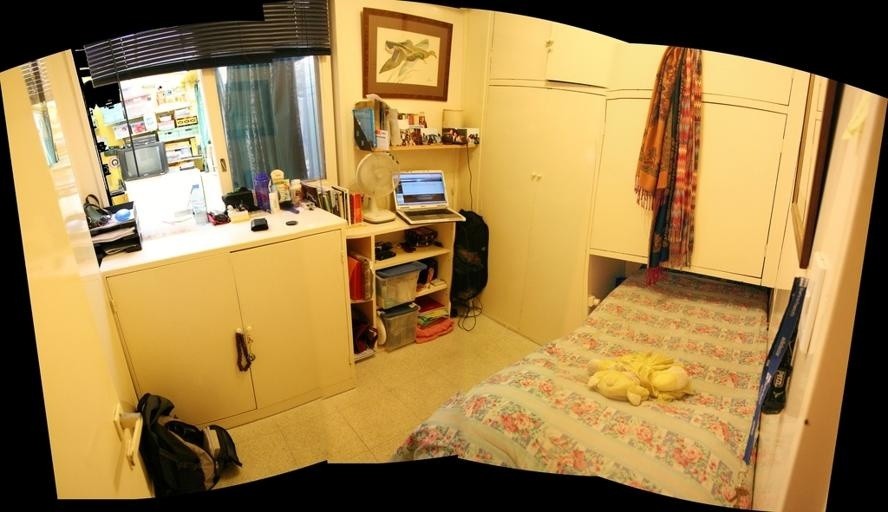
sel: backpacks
[137,392,242,497]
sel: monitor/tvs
[118,143,168,179]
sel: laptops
[389,170,467,225]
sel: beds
[383,264,778,508]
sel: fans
[354,151,402,224]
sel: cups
[406,111,416,125]
[418,110,426,125]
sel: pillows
[465,361,742,506]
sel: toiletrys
[405,128,433,146]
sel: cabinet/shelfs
[101,93,159,146]
[468,83,609,353]
[100,202,358,435]
[485,11,620,91]
[154,103,203,163]
[345,208,469,366]
[587,89,792,283]
[615,43,797,106]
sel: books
[300,180,362,225]
[201,426,221,459]
[413,295,447,316]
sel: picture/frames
[360,6,455,104]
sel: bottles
[412,127,422,144]
[253,172,269,212]
[190,183,210,227]
[289,177,303,206]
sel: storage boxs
[130,122,146,134]
[103,103,125,124]
[379,301,422,353]
[376,259,429,311]
[113,124,130,140]
[158,110,199,162]
[178,156,204,172]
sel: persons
[448,129,465,144]
